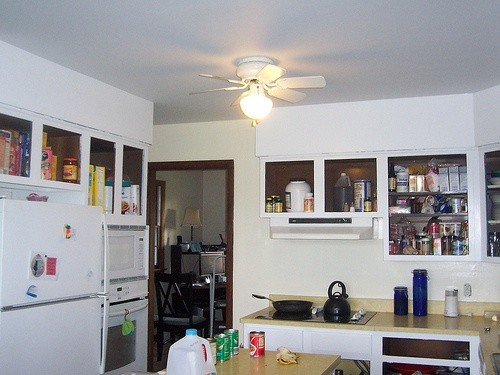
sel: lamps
[180,208,202,242]
[239,85,275,127]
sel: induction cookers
[255,309,377,325]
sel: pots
[252,294,313,313]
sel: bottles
[266,194,282,213]
[488,231,500,257]
[344,201,356,212]
[410,197,419,213]
[304,193,314,212]
[364,197,372,212]
[372,193,377,212]
[394,286,408,315]
[166,328,217,375]
[445,287,459,317]
[334,172,353,211]
[285,177,312,212]
[389,162,397,192]
[412,269,428,316]
[389,219,469,255]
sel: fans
[185,56,325,107]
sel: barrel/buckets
[353,180,372,212]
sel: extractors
[269,214,372,240]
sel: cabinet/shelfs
[84,130,147,227]
[0,102,84,192]
[478,141,500,262]
[377,329,481,375]
[385,148,478,262]
[260,152,384,217]
[246,324,374,375]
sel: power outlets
[464,285,471,296]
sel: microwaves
[101,223,148,277]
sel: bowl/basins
[390,363,437,375]
[487,177,500,185]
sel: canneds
[389,240,398,254]
[395,172,425,192]
[206,329,239,365]
[421,223,463,255]
[62,158,78,183]
[353,180,372,212]
[250,331,265,357]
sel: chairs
[154,272,211,360]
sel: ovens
[100,276,150,375]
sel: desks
[156,348,342,375]
[178,282,227,336]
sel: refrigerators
[1,198,109,375]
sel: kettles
[324,281,350,317]
